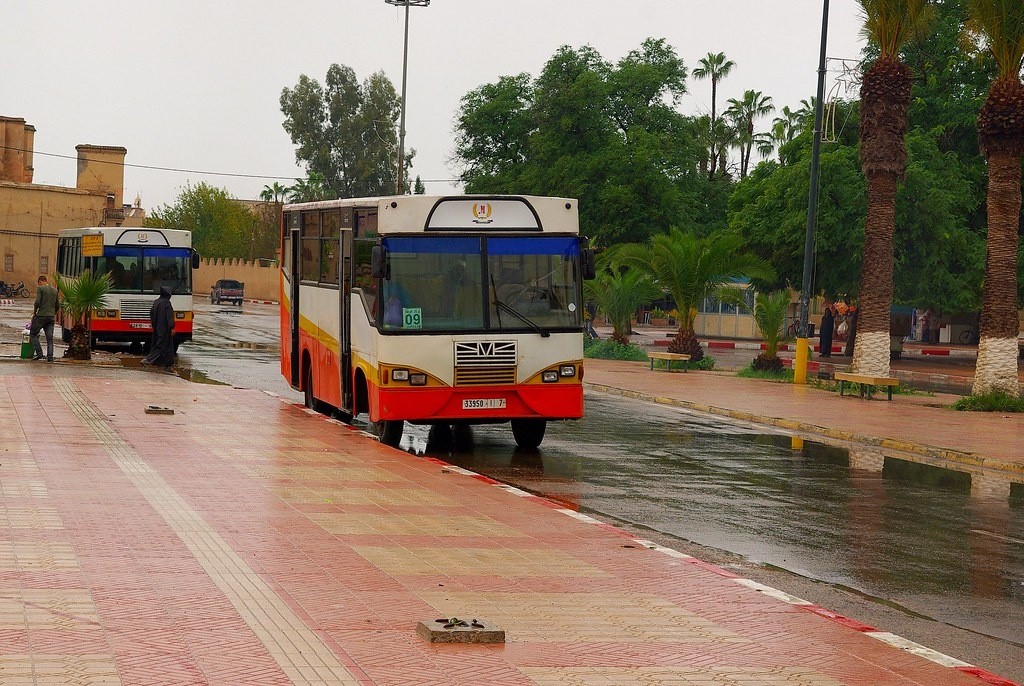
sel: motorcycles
[6,281,31,298]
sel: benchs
[647,351,692,373]
[834,372,900,402]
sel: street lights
[386,1,429,194]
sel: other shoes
[48,359,53,363]
[164,367,177,375]
[819,354,825,357]
[33,356,42,360]
[140,358,151,370]
[825,354,830,357]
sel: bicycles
[787,315,801,341]
[0,281,12,298]
[959,322,980,345]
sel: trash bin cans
[807,323,815,338]
[21,334,40,358]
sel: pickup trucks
[210,279,245,306]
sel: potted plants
[630,313,638,325]
[650,306,669,326]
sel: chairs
[496,284,532,313]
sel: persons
[140,287,177,373]
[436,261,474,306]
[96,257,124,290]
[512,269,548,299]
[819,308,834,357]
[30,276,60,362]
[127,261,156,290]
[384,280,411,325]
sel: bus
[53,228,200,353]
[280,193,595,449]
